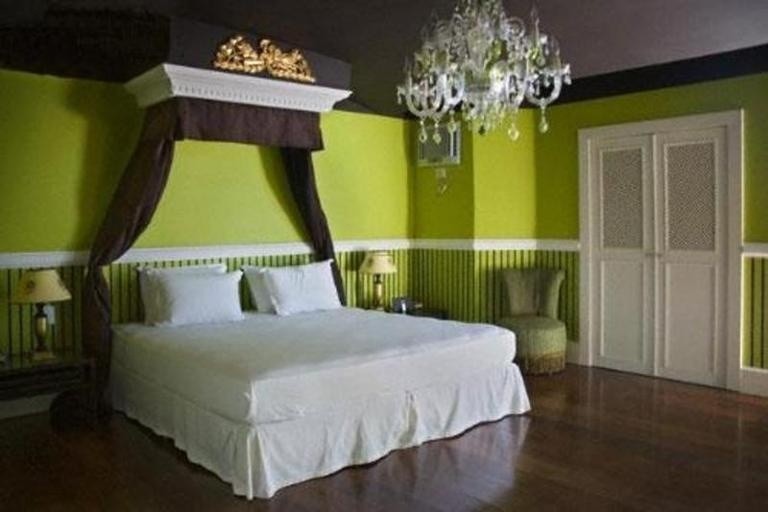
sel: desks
[0,356,96,422]
[384,310,446,320]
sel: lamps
[396,0,572,145]
[9,269,72,359]
[359,252,398,311]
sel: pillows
[241,264,274,312]
[148,269,245,328]
[135,263,226,325]
[259,258,341,316]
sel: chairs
[496,268,567,376]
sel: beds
[109,307,533,500]
